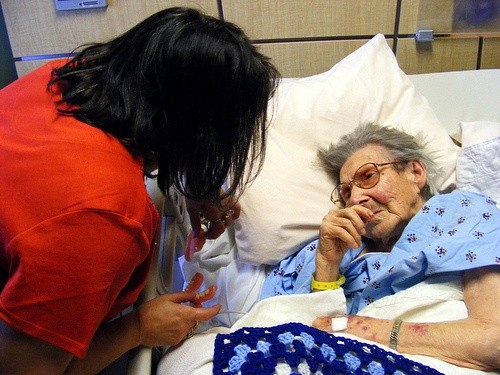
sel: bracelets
[310,272,345,291]
[389,320,402,352]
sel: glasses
[329,159,417,208]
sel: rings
[184,328,193,339]
[194,321,198,329]
[223,208,234,219]
[210,217,222,223]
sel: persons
[309,121,500,372]
[0,6,282,375]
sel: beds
[127,68,500,374]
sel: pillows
[213,34,458,265]
